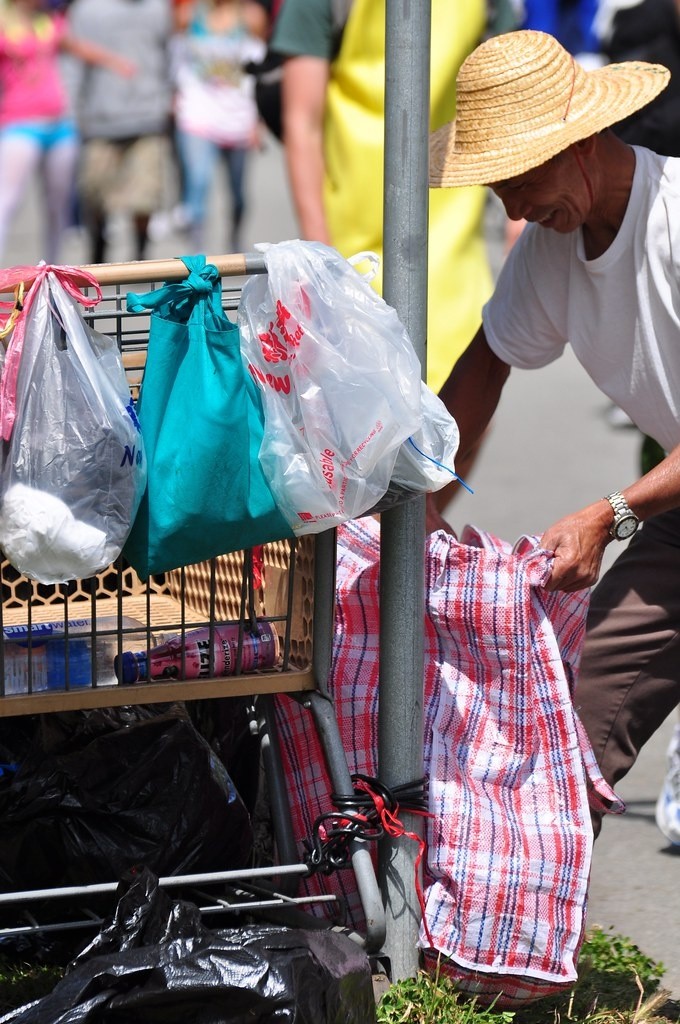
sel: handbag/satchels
[120,254,307,574]
[237,239,423,538]
[245,29,344,140]
[0,264,145,585]
[350,250,461,518]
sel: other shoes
[655,725,680,846]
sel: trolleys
[0,253,397,1013]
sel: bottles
[114,621,279,684]
[3,616,178,694]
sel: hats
[427,29,672,188]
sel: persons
[373,28,680,865]
[1,0,138,263]
[266,0,514,400]
[166,1,267,255]
[56,1,182,266]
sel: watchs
[604,491,639,542]
